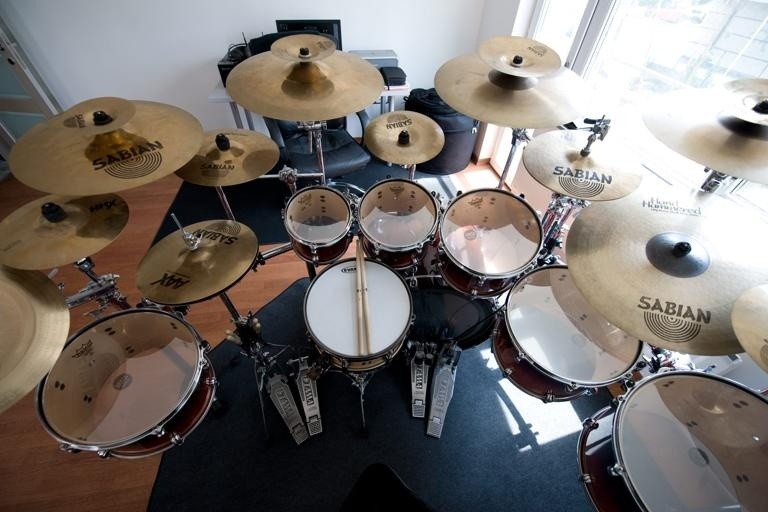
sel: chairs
[248,31,371,196]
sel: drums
[491,263,643,403]
[395,240,497,350]
[34,307,218,460]
[281,185,353,265]
[302,257,416,373]
[357,176,442,270]
[576,370,768,512]
[436,188,544,300]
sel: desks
[207,73,411,168]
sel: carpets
[145,278,615,512]
[138,147,460,247]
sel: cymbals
[174,128,280,187]
[225,49,385,122]
[477,35,562,79]
[642,87,767,185]
[716,77,768,127]
[8,100,204,197]
[0,193,129,271]
[731,284,768,374]
[135,220,259,305]
[1,264,70,412]
[565,180,768,355]
[60,96,136,136]
[522,128,643,201]
[364,110,444,165]
[434,52,595,130]
[271,34,336,63]
